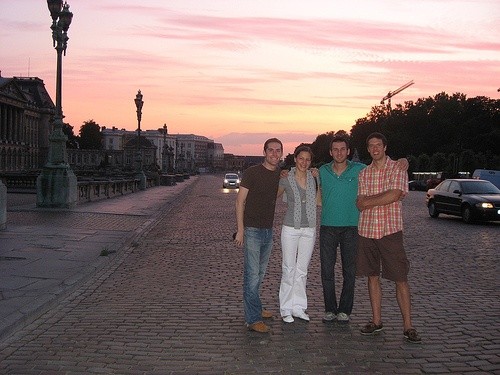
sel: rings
[405,166,406,169]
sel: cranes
[380,80,415,107]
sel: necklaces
[294,173,306,202]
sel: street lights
[133,88,144,162]
[162,123,168,156]
[46,0,74,169]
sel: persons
[354,132,421,343]
[278,136,409,324]
[276,146,320,323]
[234,137,320,333]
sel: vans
[472,169,500,190]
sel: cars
[425,179,500,225]
[408,179,442,193]
[223,173,240,189]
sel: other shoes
[322,312,337,322]
[282,315,294,323]
[337,312,350,322]
[360,320,384,335]
[403,327,422,343]
[262,310,272,317]
[247,321,270,332]
[293,310,311,322]
